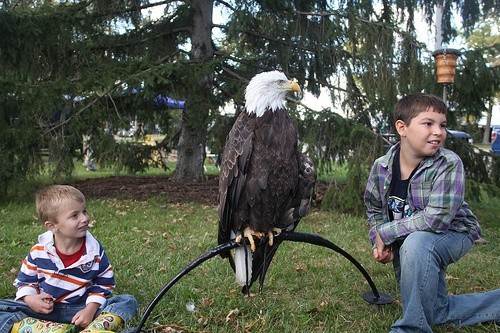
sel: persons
[0,185,139,332]
[363,93,500,333]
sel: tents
[67,79,187,111]
